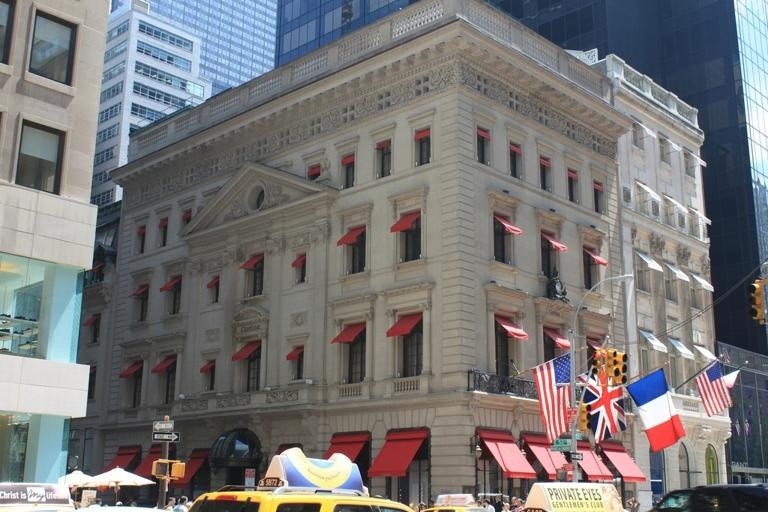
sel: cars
[415,493,491,512]
[183,446,418,511]
[646,482,767,512]
[518,483,627,512]
[0,481,79,512]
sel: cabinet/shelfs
[0,316,39,357]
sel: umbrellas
[59,465,156,502]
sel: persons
[89,498,102,507]
[162,496,188,512]
[115,496,137,506]
[626,497,640,512]
[476,495,523,512]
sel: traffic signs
[153,420,176,432]
[152,432,181,442]
[551,431,587,462]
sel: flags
[734,416,742,437]
[744,417,750,437]
[532,352,572,444]
[695,359,740,417]
[576,363,627,445]
[625,368,687,453]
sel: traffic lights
[606,348,620,378]
[612,351,628,385]
[152,461,169,478]
[748,279,766,323]
[579,402,592,433]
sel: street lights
[570,272,635,481]
